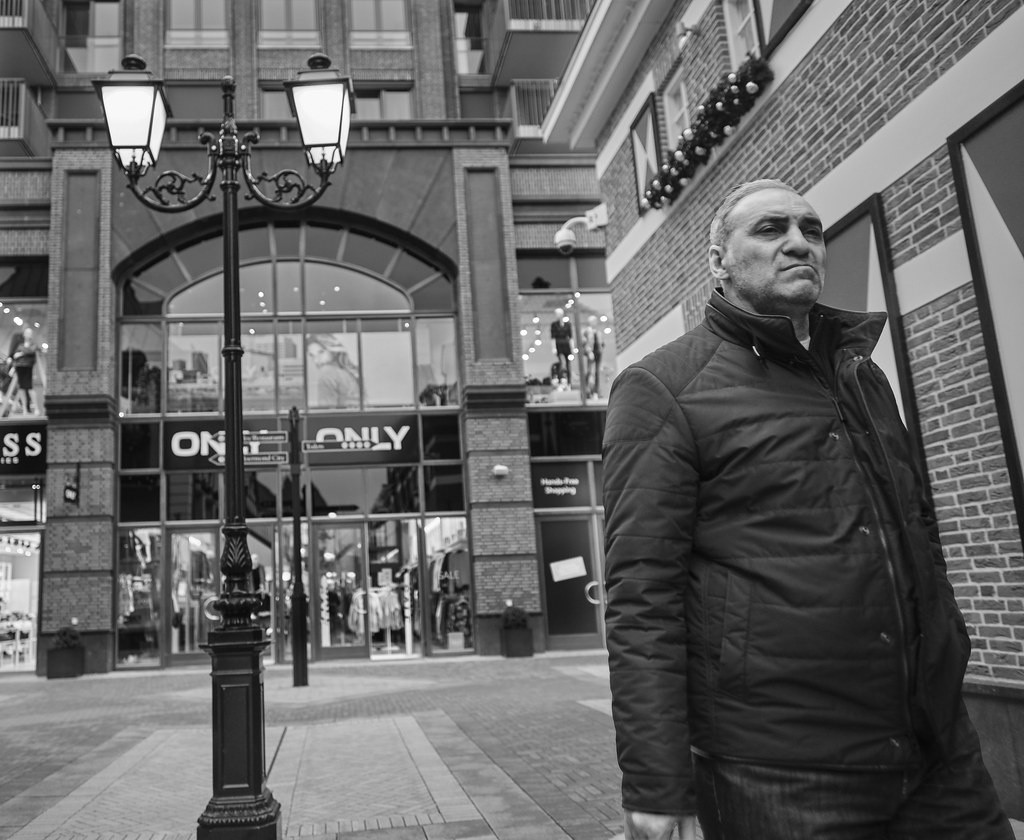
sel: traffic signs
[300,439,379,453]
[208,450,290,467]
[210,430,290,445]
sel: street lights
[91,52,359,840]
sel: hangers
[453,597,470,606]
[452,543,468,554]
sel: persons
[251,554,359,645]
[580,315,605,400]
[0,328,40,416]
[551,308,574,390]
[601,177,1016,839]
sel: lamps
[64,480,80,504]
[492,464,509,475]
